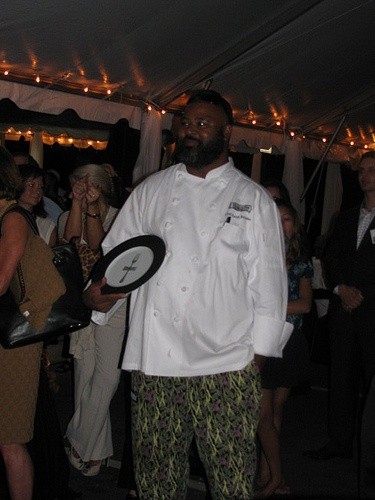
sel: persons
[304,146,375,460]
[0,149,69,500]
[57,163,128,477]
[253,181,313,500]
[0,145,63,500]
[81,88,295,500]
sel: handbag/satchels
[0,204,92,348]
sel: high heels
[82,454,110,476]
[69,445,83,470]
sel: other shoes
[304,440,354,461]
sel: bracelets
[84,212,101,219]
